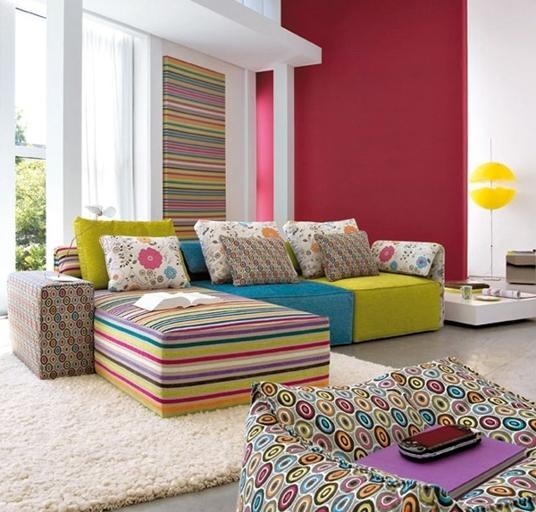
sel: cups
[459,286,472,300]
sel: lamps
[471,138,516,282]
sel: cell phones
[395,419,481,460]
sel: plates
[475,296,500,301]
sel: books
[354,425,526,500]
[482,288,536,299]
[132,292,220,312]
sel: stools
[239,360,536,512]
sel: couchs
[6,242,446,421]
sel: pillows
[282,218,359,278]
[372,239,440,276]
[100,234,190,291]
[314,231,379,281]
[74,217,190,289]
[222,235,300,286]
[194,220,284,285]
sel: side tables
[444,291,536,328]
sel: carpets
[3,319,399,511]
